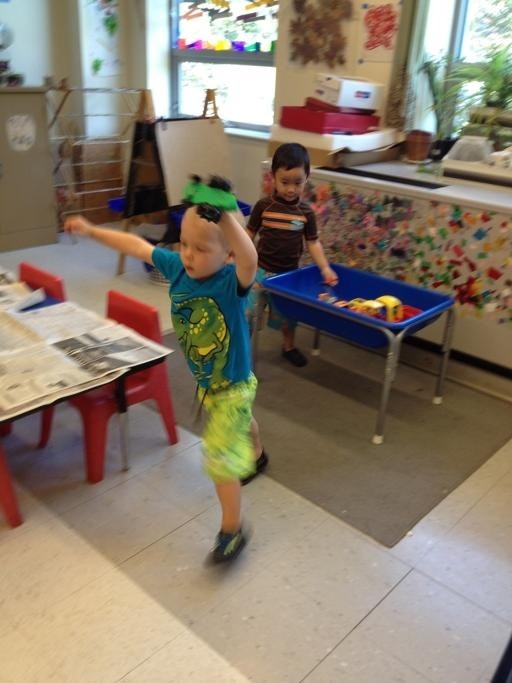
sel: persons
[63,206,268,570]
[244,143,339,368]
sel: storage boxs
[267,72,405,170]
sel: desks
[0,270,159,529]
[259,153,512,400]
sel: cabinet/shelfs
[78,141,125,222]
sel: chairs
[15,255,178,484]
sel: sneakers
[212,527,246,563]
[282,348,308,366]
[239,448,268,486]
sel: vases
[406,130,430,158]
